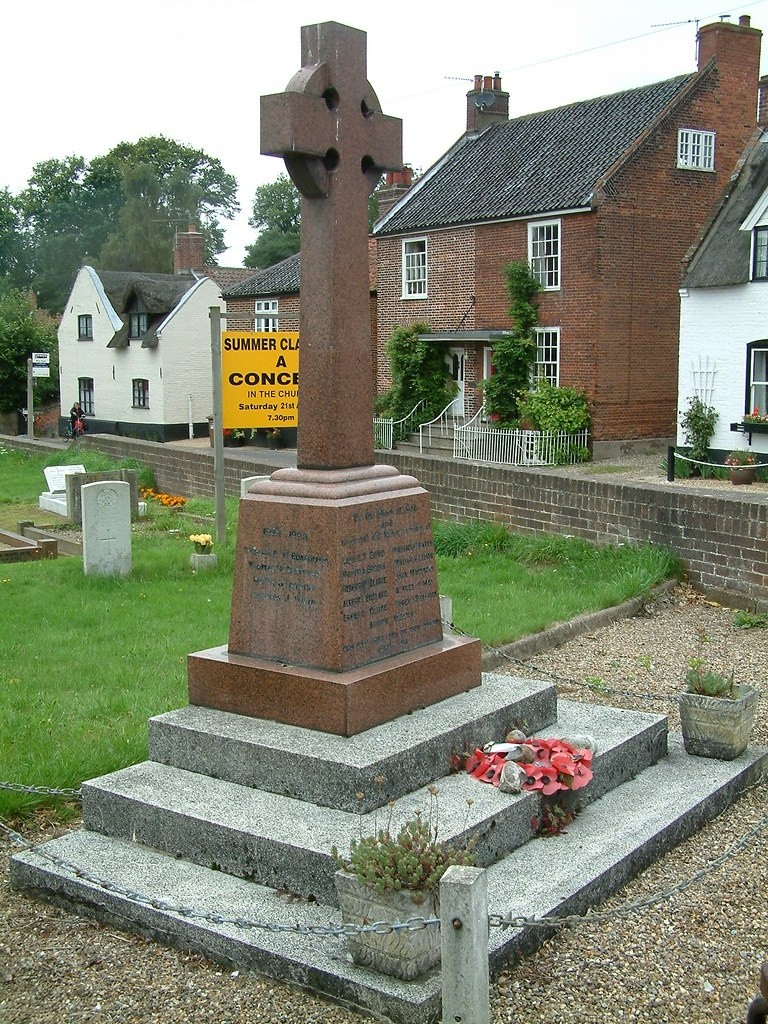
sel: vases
[269,438,281,450]
[731,470,754,486]
[190,552,217,571]
[744,423,768,434]
[223,438,243,447]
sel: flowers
[267,428,281,439]
[189,534,214,554]
[223,428,245,441]
[724,451,759,471]
[743,407,768,423]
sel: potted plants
[669,395,720,476]
[332,772,495,980]
[678,625,758,761]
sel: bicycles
[61,413,85,443]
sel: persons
[70,402,85,440]
